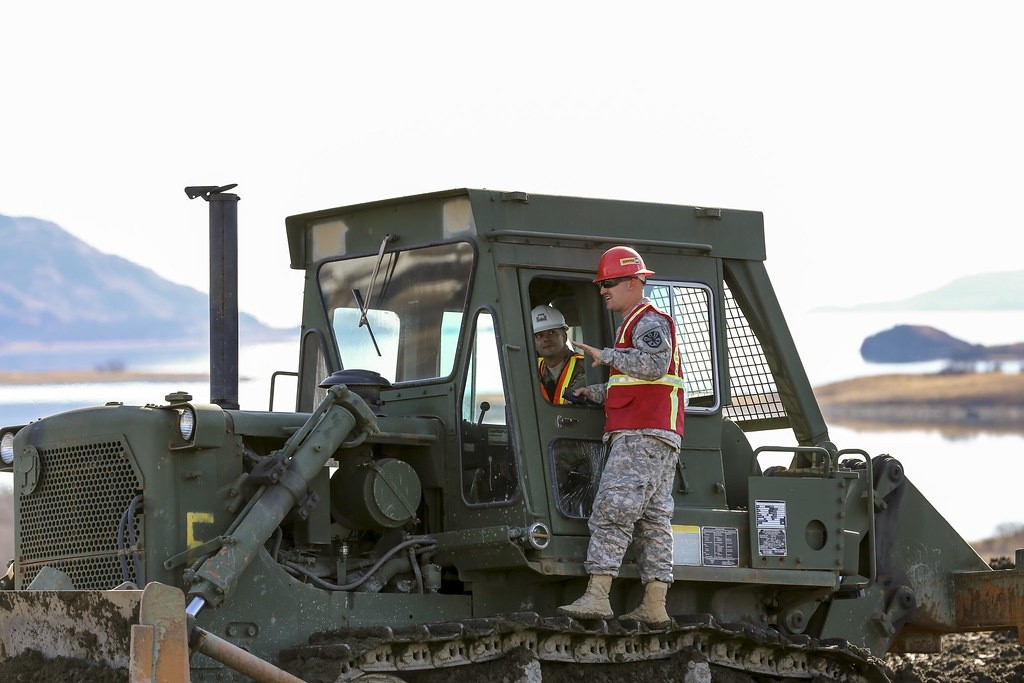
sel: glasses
[598,275,636,288]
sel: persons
[571,245,686,629]
[531,304,585,405]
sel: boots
[618,581,671,630]
[558,573,614,621]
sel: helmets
[530,304,569,334]
[592,245,656,283]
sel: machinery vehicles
[0,182,1024,683]
